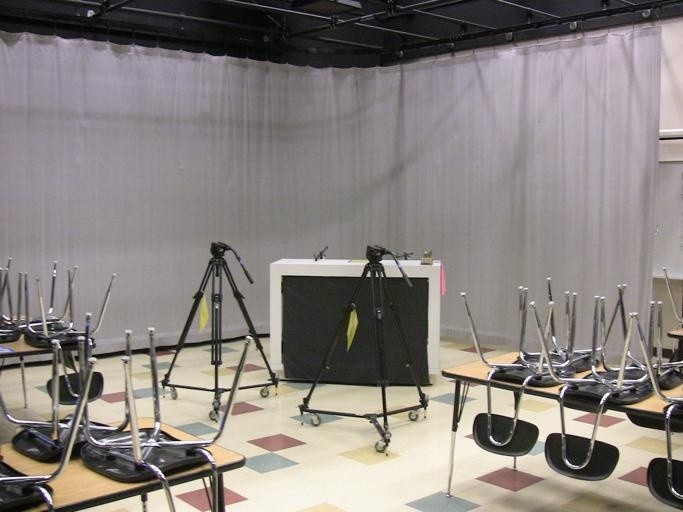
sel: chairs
[460,287,578,458]
[632,311,683,510]
[528,295,656,481]
[0,310,133,463]
[24,268,117,406]
[0,257,22,344]
[76,326,253,512]
[599,283,683,391]
[518,277,627,373]
[0,357,97,512]
[5,260,79,335]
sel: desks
[0,416,246,512]
[441,351,683,499]
[267,257,442,385]
[0,333,97,410]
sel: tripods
[298,243,428,446]
[159,241,279,412]
